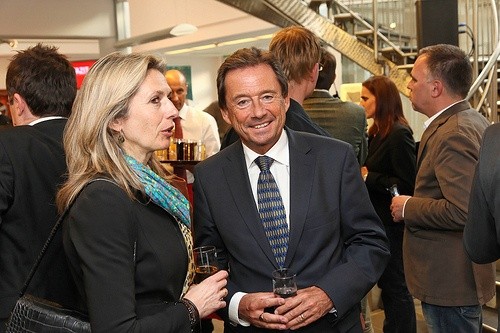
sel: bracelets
[178,298,199,329]
[363,172,369,182]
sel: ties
[254,156,290,271]
[173,116,183,139]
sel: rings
[392,212,396,218]
[259,313,263,321]
[224,288,228,297]
[301,314,307,320]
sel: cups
[272,268,298,309]
[154,139,206,163]
[192,246,224,301]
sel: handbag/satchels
[4,179,141,333]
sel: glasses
[318,62,324,71]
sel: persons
[56,52,229,333]
[390,44,492,333]
[0,43,80,333]
[359,75,417,333]
[202,100,232,145]
[220,25,334,150]
[153,69,221,246]
[0,103,11,125]
[193,46,391,333]
[463,122,500,264]
[302,47,368,167]
